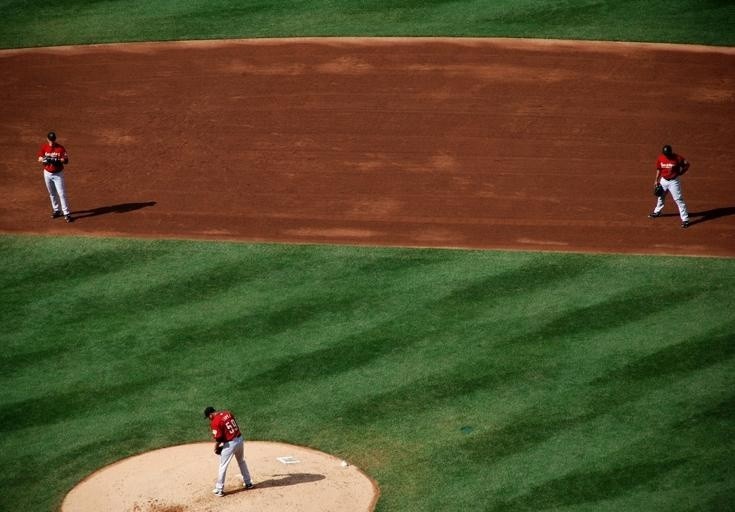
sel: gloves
[42,156,60,164]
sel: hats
[663,145,672,155]
[48,132,55,140]
[204,407,216,419]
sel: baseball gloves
[653,182,664,197]
[215,446,223,456]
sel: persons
[35,132,73,223]
[204,406,254,497]
[647,144,693,228]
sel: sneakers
[51,211,63,218]
[648,211,663,218]
[64,215,72,223]
[680,221,689,228]
[243,483,253,488]
[213,489,224,497]
